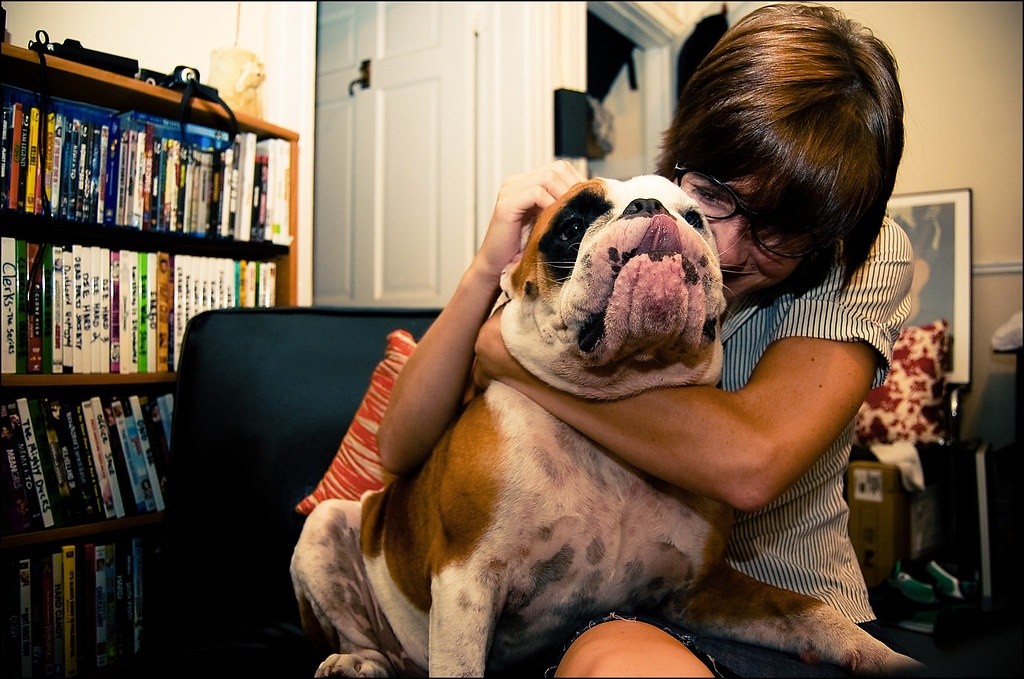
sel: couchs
[843,319,956,587]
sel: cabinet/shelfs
[0,39,301,677]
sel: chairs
[159,306,467,677]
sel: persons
[375,3,915,678]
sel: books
[0,81,293,679]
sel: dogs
[288,174,932,679]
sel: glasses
[675,160,816,256]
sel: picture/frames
[880,187,974,387]
